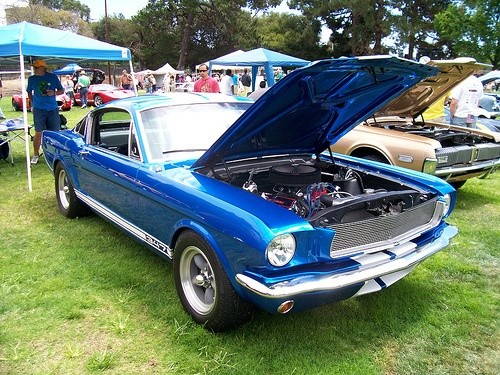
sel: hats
[30,60,47,68]
[80,71,87,75]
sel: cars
[431,69,500,132]
[248,56,500,190]
[40,52,460,334]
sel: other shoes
[81,104,87,109]
[31,155,40,165]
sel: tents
[53,62,83,84]
[0,21,138,191]
[148,63,185,93]
[196,50,281,76]
[208,47,311,92]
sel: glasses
[199,69,207,73]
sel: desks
[0,122,33,166]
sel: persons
[193,64,220,93]
[421,91,452,123]
[26,60,64,164]
[208,68,293,100]
[77,70,90,109]
[448,74,484,129]
[134,73,202,94]
[483,81,500,92]
[64,75,75,106]
[119,70,133,90]
[0,80,5,119]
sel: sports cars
[11,89,73,113]
[70,68,135,108]
